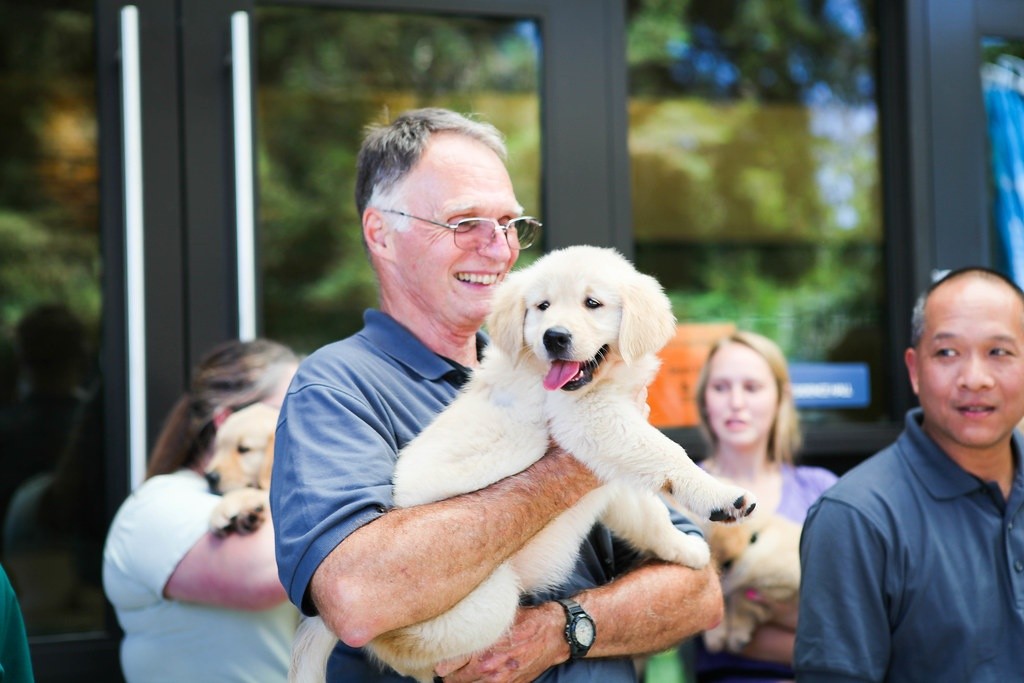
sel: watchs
[553,597,596,663]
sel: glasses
[376,206,543,252]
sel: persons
[1,301,106,633]
[269,107,1024,683]
[0,563,35,683]
[103,337,301,683]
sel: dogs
[368,245,759,683]
[665,481,809,652]
[197,401,281,538]
